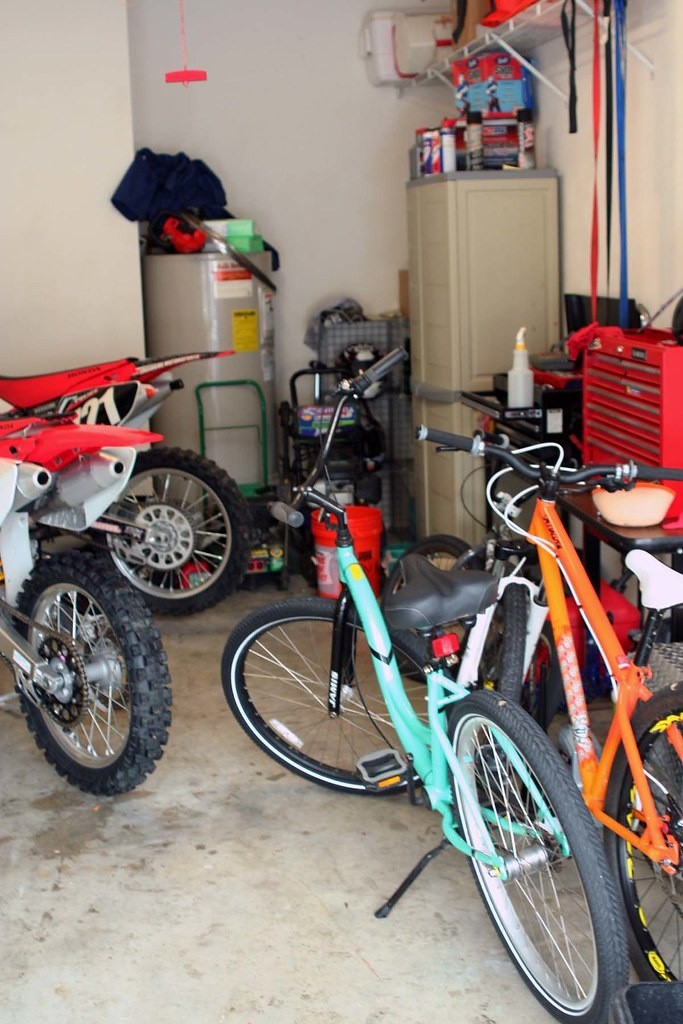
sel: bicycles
[221,346,683,1024]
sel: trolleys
[195,379,292,595]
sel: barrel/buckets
[311,505,384,601]
[215,496,290,588]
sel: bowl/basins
[591,483,675,527]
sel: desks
[483,452,683,642]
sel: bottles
[466,112,484,171]
[517,106,535,170]
[416,118,460,176]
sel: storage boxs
[291,406,359,438]
[457,117,519,169]
[454,55,483,112]
[202,235,263,254]
[201,218,256,237]
[483,52,538,114]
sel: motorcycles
[0,348,234,798]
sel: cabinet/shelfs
[405,168,560,552]
